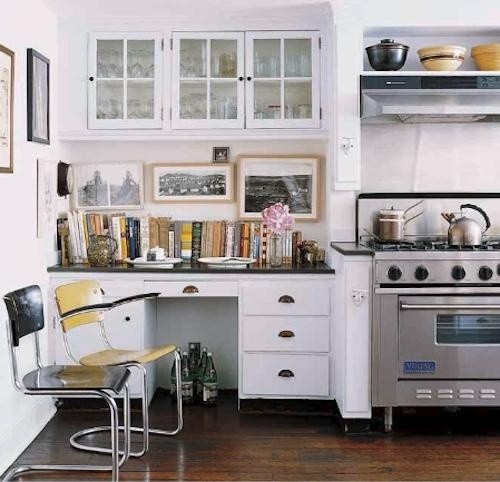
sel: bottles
[254,100,311,118]
[169,346,219,408]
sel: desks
[49,256,336,415]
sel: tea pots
[441,203,491,246]
[85,232,118,267]
[296,240,319,264]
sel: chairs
[1,284,136,480]
[53,281,189,459]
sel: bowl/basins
[470,44,500,70]
[417,44,468,71]
[362,37,409,70]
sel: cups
[218,52,310,77]
[150,246,169,262]
[94,91,214,120]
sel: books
[57,209,302,266]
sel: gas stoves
[358,234,500,285]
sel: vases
[267,226,284,266]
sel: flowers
[260,204,295,234]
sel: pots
[377,201,424,240]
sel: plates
[197,255,258,271]
[127,255,183,270]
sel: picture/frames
[234,153,324,222]
[35,158,59,240]
[68,160,145,210]
[147,161,235,205]
[0,43,15,177]
[24,47,52,147]
[212,146,230,163]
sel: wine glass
[97,46,197,77]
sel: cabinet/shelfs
[87,29,165,130]
[169,29,320,126]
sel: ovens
[372,288,499,408]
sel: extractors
[360,75,500,125]
